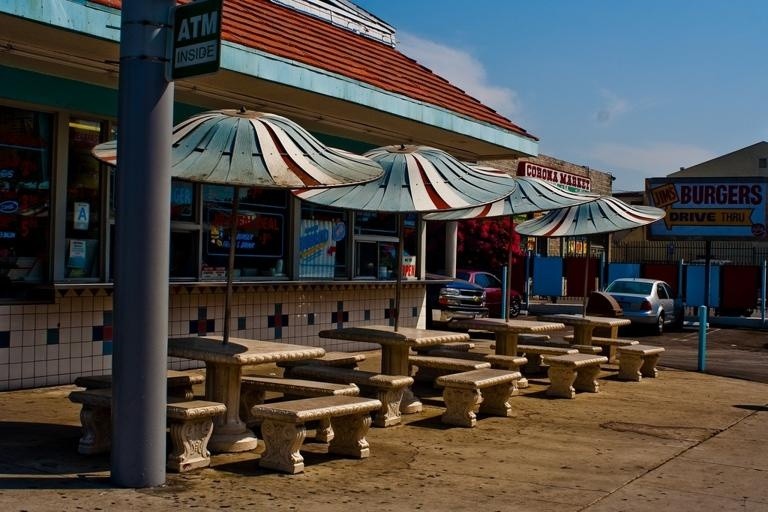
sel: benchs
[409,353,492,394]
[68,388,227,476]
[291,364,414,429]
[251,393,383,474]
[490,333,642,374]
[614,344,665,382]
[540,352,610,400]
[275,350,368,380]
[437,369,523,428]
[75,370,206,403]
[240,375,359,443]
[414,341,532,388]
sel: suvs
[379,246,489,332]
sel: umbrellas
[515,195,667,318]
[290,144,517,332]
[92,106,385,345]
[421,176,601,322]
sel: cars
[603,277,674,335]
[455,269,523,318]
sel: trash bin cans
[586,292,624,363]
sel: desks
[545,313,631,346]
[166,334,326,455]
[450,317,566,388]
[318,327,469,415]
